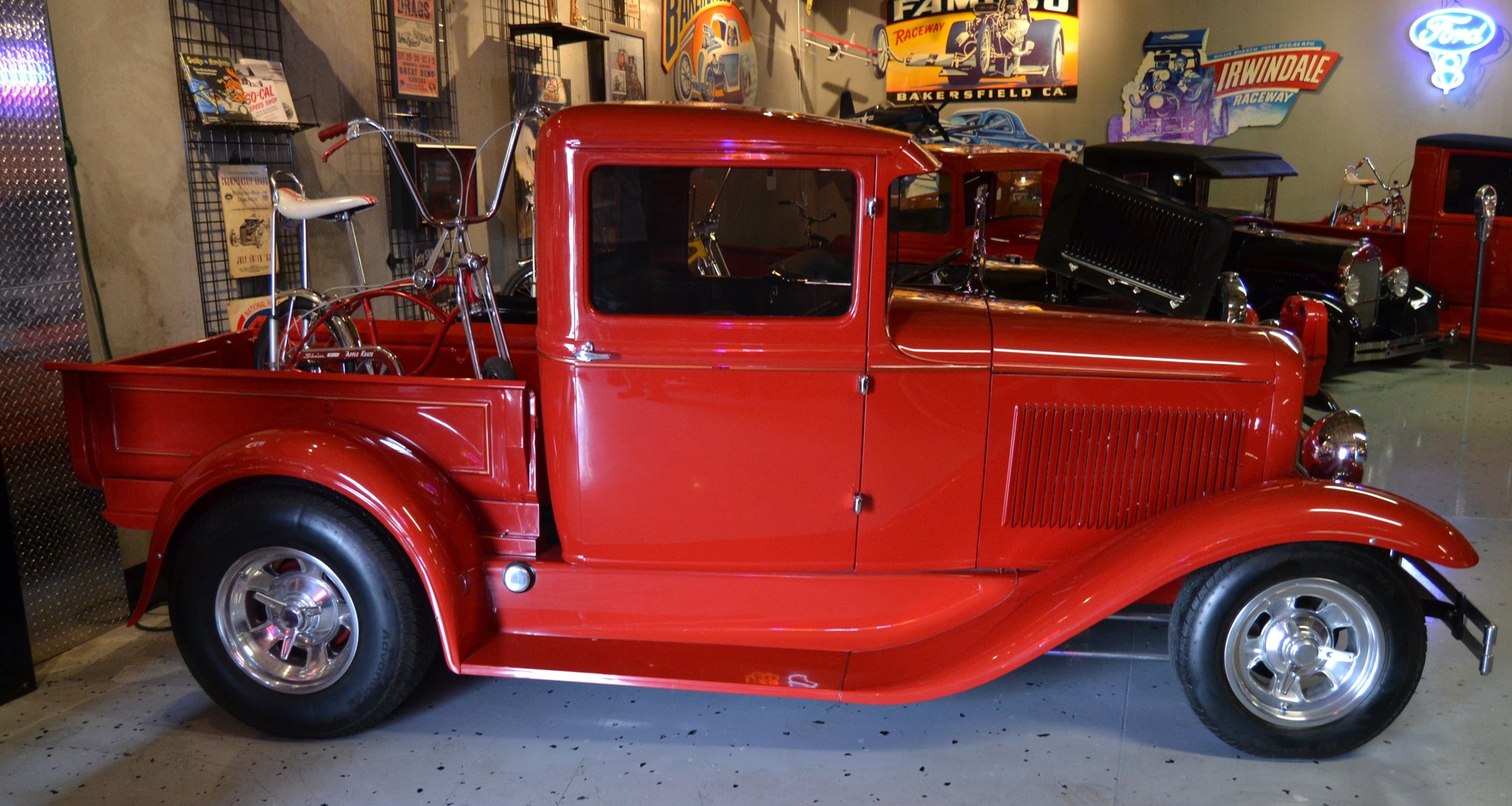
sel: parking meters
[1449,185,1497,372]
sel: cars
[929,109,1049,152]
[1084,142,1448,373]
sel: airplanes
[804,32,871,67]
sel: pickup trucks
[1285,135,1512,352]
[45,97,1499,761]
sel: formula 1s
[1105,28,1230,146]
[941,1,1066,85]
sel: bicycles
[1329,154,1415,231]
[253,99,555,381]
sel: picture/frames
[603,19,647,102]
